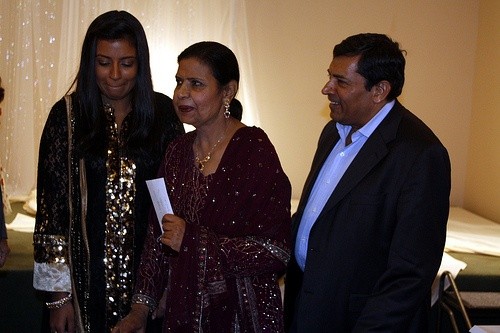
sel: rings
[113,326,118,329]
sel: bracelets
[45,293,73,313]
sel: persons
[111,41,292,333]
[283,33,452,333]
[0,76,9,268]
[33,10,186,333]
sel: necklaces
[193,116,232,173]
